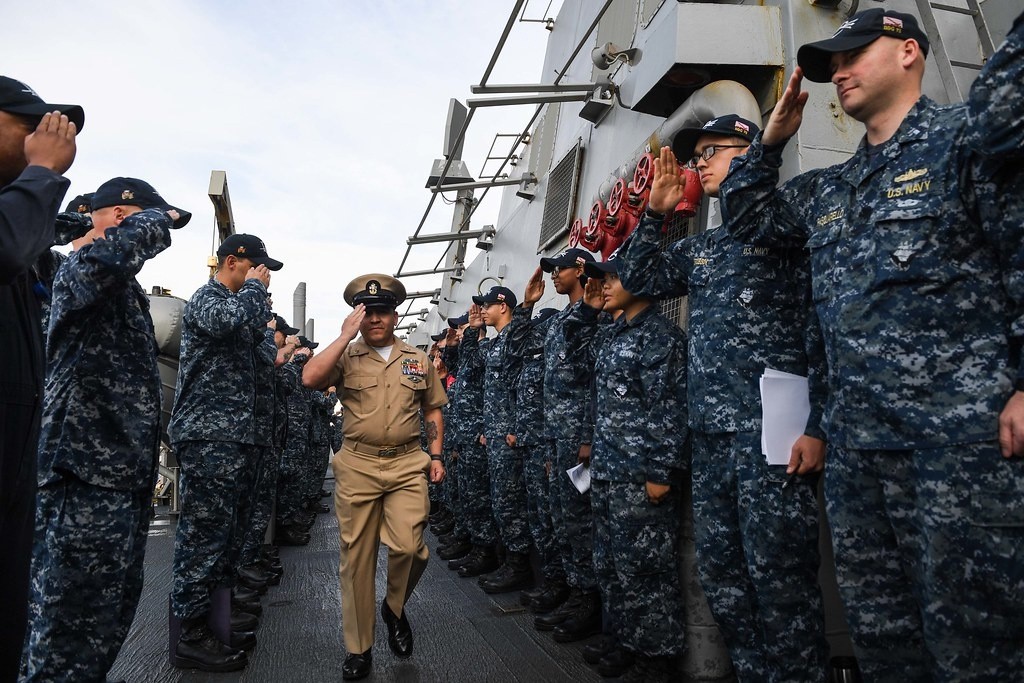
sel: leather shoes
[381,598,413,658]
[342,647,371,681]
[425,506,693,683]
[174,489,333,672]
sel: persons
[718,7,1024,683]
[617,115,828,683]
[302,274,449,680]
[0,77,343,683]
[969,9,1024,158]
[419,285,570,613]
[506,247,614,643]
[563,246,688,683]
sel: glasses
[550,264,577,275]
[692,144,751,164]
[483,301,502,309]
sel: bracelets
[645,202,665,220]
[430,454,442,460]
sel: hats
[797,7,930,83]
[217,234,284,272]
[671,113,760,161]
[275,316,300,335]
[584,245,619,279]
[531,308,560,324]
[343,274,406,308]
[296,336,320,349]
[90,177,192,229]
[472,285,518,310]
[447,310,488,333]
[0,76,84,134]
[580,274,589,289]
[430,328,450,341]
[65,193,90,214]
[540,248,596,273]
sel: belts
[342,437,420,457]
